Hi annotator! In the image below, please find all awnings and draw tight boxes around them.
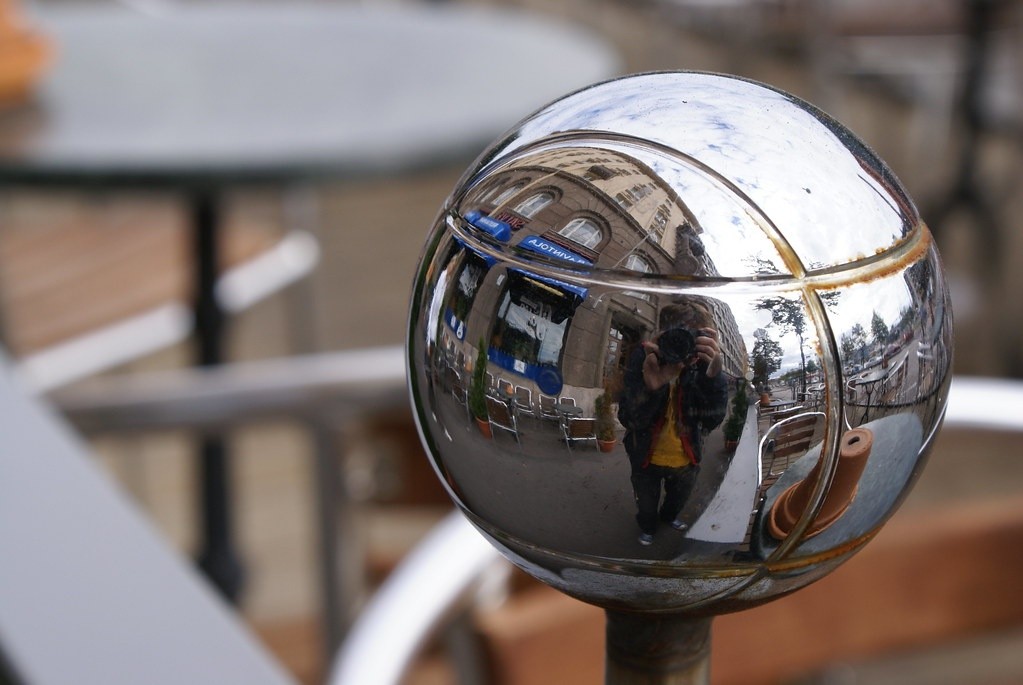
[506,234,592,303]
[454,210,512,269]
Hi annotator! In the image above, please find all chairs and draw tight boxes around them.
[427,323,598,456]
[751,353,909,515]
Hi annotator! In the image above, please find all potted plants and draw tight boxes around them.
[596,392,620,451]
[722,385,749,449]
[469,335,493,439]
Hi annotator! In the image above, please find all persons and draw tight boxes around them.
[616,303,728,545]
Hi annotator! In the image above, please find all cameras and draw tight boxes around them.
[657,327,710,358]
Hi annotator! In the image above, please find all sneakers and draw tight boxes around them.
[670,518,688,531]
[639,532,655,546]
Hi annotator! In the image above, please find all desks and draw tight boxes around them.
[766,400,797,410]
[855,360,897,423]
